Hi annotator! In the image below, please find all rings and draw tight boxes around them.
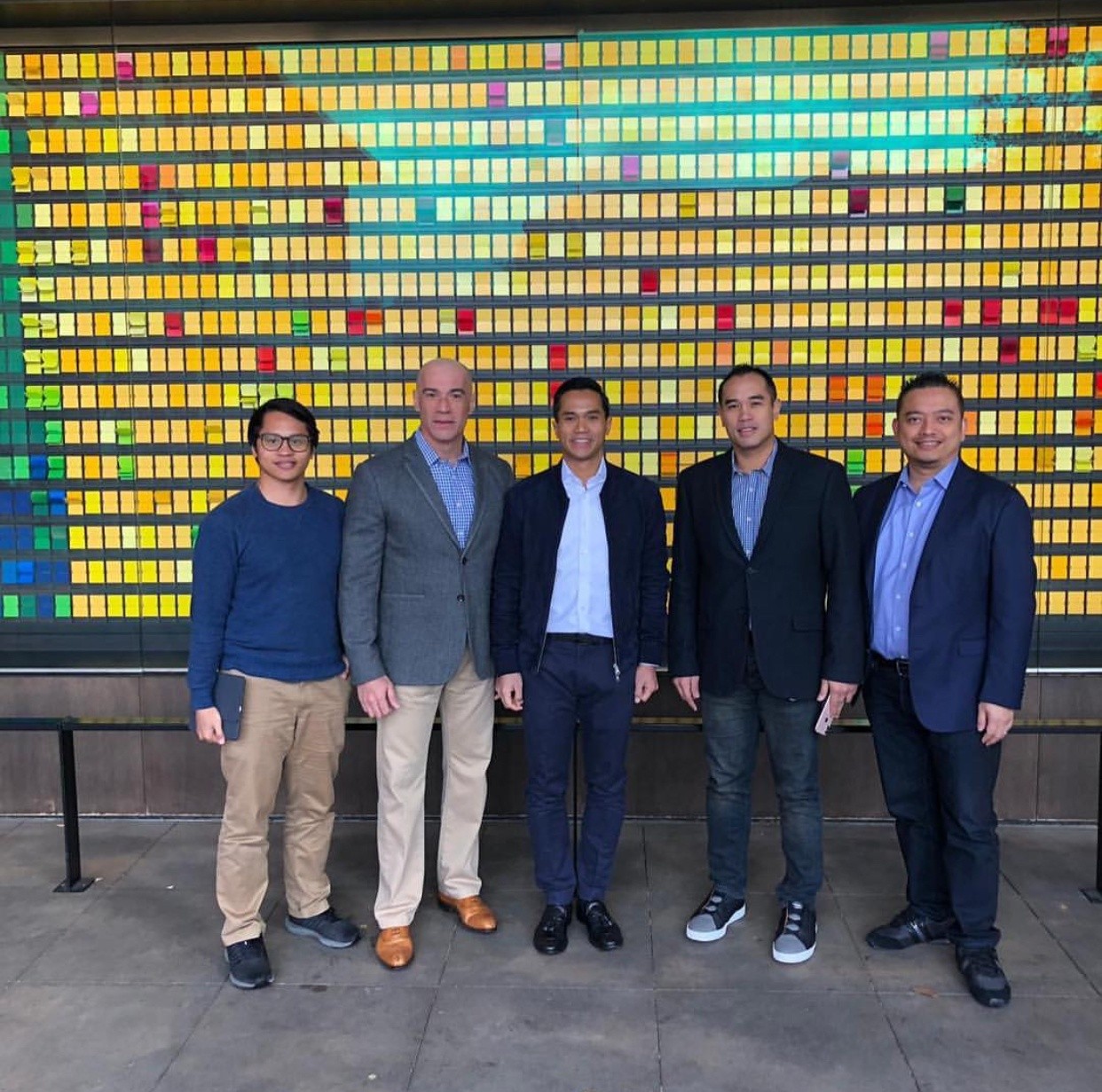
[1004,731,1007,735]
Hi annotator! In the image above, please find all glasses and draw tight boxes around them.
[256,433,313,453]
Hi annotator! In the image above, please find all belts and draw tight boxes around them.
[872,651,911,677]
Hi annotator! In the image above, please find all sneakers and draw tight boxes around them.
[956,944,1009,1008]
[867,910,960,949]
[223,938,274,990]
[686,887,747,941]
[284,907,360,949]
[772,900,819,962]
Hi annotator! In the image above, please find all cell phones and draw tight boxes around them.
[814,692,834,736]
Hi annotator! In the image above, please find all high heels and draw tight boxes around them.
[533,902,571,955]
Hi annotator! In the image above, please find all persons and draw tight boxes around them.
[852,372,1038,1008]
[665,364,865,964]
[337,357,516,968]
[487,377,668,954]
[187,398,361,989]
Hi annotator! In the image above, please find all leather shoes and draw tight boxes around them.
[374,924,412,969]
[438,890,496,933]
[576,899,622,950]
